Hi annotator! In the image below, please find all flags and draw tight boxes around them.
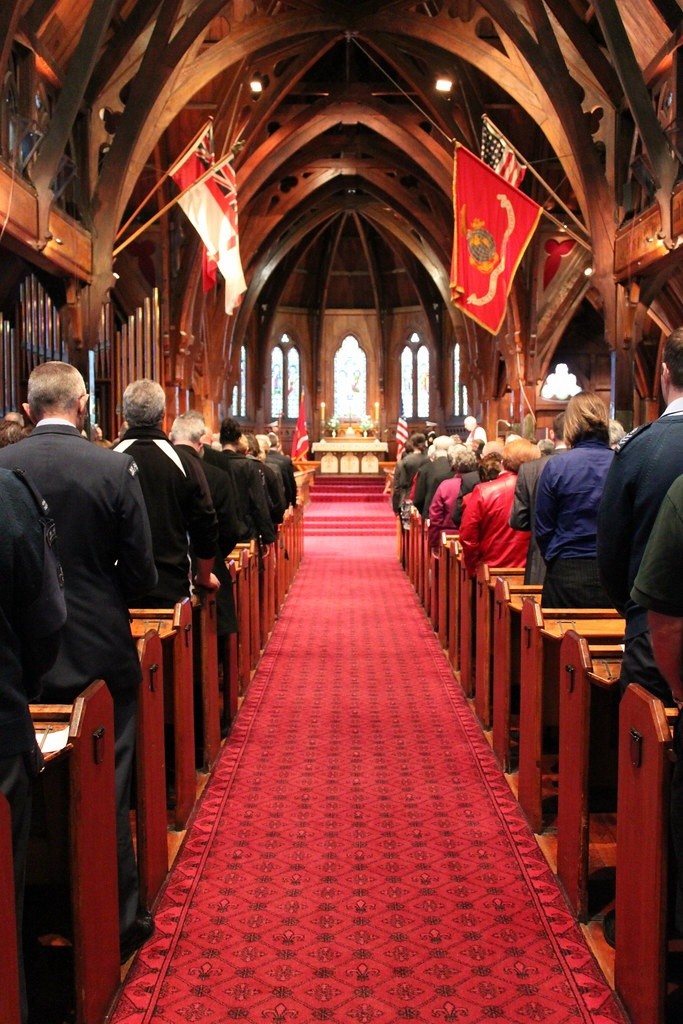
[169,123,217,295]
[481,128,527,187]
[176,154,247,314]
[451,144,544,336]
[396,396,409,459]
[291,394,308,471]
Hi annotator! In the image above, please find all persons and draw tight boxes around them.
[464,416,487,445]
[393,323,683,1024]
[0,360,300,1024]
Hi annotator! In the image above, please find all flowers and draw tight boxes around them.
[326,417,340,430]
[359,414,374,431]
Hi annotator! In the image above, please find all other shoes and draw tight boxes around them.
[120,905,155,965]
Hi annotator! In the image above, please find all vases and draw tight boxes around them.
[363,430,368,438]
[345,427,355,437]
[331,429,336,438]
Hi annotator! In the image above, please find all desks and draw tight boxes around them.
[0,495,683,1024]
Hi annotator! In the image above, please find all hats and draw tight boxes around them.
[269,421,278,427]
[425,421,437,427]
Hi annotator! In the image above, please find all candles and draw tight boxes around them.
[375,402,380,420]
[321,402,325,419]
